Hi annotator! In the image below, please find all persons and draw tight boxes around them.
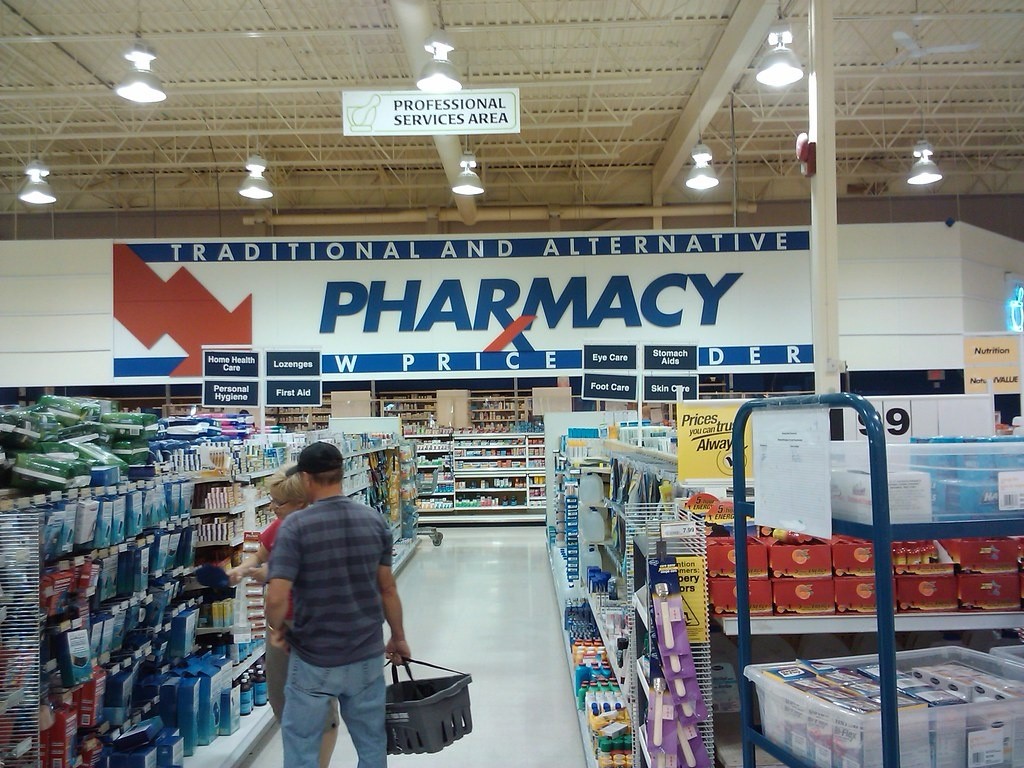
[265,441,410,768]
[226,462,340,767]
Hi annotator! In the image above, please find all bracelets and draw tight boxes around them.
[267,624,275,632]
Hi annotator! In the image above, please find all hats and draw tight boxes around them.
[287,441,344,477]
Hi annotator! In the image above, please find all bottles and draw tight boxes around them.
[212,633,227,661]
[248,669,256,682]
[254,670,267,706]
[572,637,633,768]
[240,679,251,716]
[226,632,252,666]
[254,665,266,678]
[242,673,249,679]
[244,676,254,711]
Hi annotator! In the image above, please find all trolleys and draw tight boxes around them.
[418,469,443,546]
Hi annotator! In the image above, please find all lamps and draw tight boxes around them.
[18,0,942,206]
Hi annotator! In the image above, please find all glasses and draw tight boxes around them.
[267,493,294,509]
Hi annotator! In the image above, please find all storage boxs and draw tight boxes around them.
[0,393,1024,768]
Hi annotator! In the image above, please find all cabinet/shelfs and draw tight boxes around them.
[732,391,1024,768]
[545,410,1024,768]
[382,389,527,429]
[451,433,546,515]
[404,433,454,515]
[266,394,383,428]
[0,417,422,768]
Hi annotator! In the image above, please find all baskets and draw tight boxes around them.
[383,657,473,755]
